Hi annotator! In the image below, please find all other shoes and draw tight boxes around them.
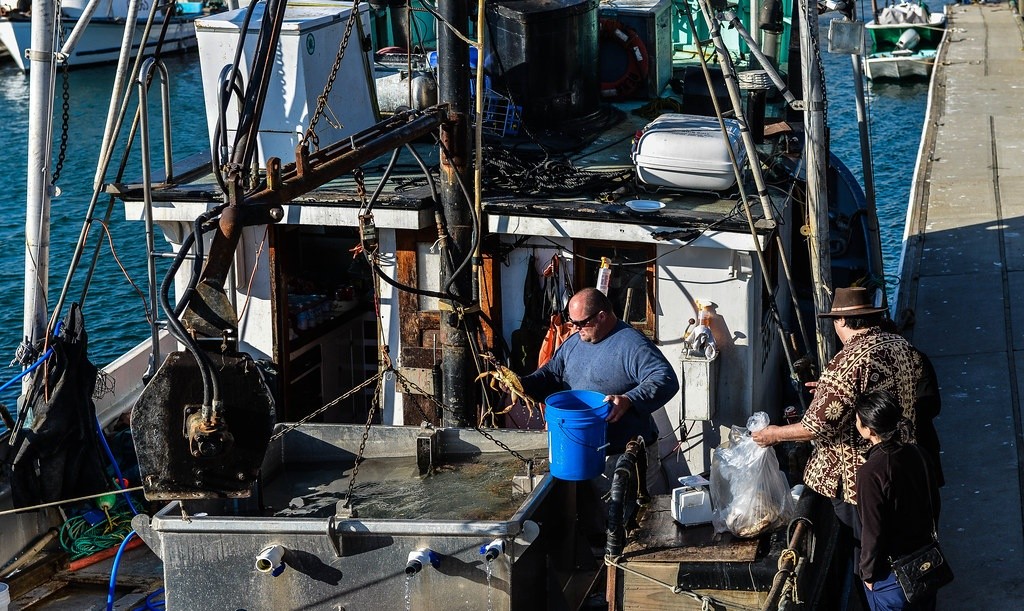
[590,547,619,557]
[587,589,622,606]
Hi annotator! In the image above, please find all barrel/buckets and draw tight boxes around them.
[0,582,10,611]
[544,389,611,480]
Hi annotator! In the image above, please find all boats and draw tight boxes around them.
[0,0,228,72]
[861,48,939,79]
[864,0,947,54]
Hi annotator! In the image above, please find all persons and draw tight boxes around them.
[499,287,680,556]
[753,287,946,610]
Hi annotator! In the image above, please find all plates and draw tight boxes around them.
[625,200,666,212]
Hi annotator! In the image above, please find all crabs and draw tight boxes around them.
[726,489,790,539]
[475,351,536,425]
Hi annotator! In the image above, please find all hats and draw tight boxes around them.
[818,287,888,318]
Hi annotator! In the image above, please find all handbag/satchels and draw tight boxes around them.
[709,411,795,538]
[891,540,954,603]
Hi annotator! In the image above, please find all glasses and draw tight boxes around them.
[572,312,599,330]
